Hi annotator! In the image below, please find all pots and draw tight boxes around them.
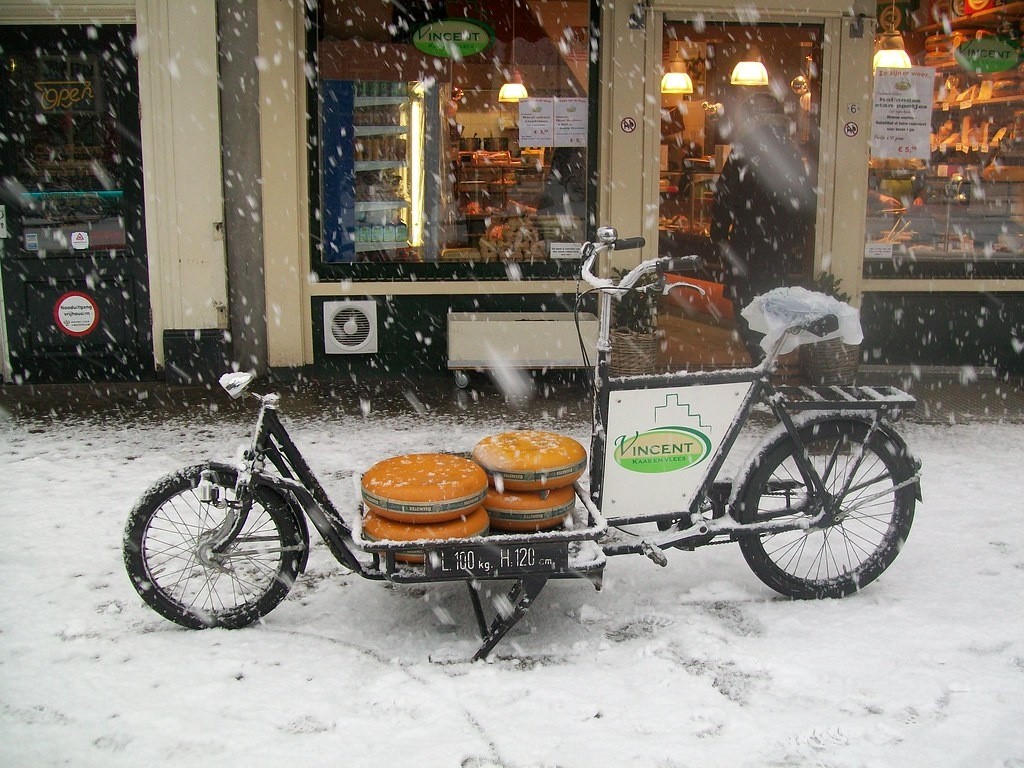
[484,138,508,151]
[460,137,480,151]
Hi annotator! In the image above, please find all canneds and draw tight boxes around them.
[353,79,409,161]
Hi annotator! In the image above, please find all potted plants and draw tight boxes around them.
[775,275,863,386]
[603,266,667,377]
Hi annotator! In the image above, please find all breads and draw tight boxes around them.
[479,217,547,262]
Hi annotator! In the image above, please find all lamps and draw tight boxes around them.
[872,0,912,69]
[498,0,527,102]
[661,39,694,94]
[731,47,768,86]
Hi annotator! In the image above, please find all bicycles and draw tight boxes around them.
[122,227,924,667]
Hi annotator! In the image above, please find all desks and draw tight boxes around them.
[688,173,721,228]
[871,163,1024,252]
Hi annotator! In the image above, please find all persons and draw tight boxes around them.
[708,92,811,368]
[534,146,586,313]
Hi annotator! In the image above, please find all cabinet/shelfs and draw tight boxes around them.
[914,0,1024,158]
[453,163,545,247]
[353,96,411,252]
